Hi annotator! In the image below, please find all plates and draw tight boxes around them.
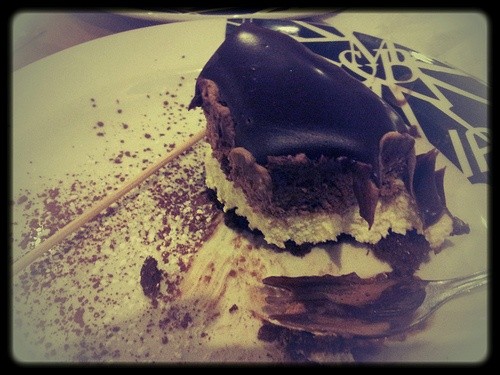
[13,17,488,363]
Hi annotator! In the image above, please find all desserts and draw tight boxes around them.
[186,23,468,362]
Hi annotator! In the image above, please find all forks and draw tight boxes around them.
[261,271,487,339]
